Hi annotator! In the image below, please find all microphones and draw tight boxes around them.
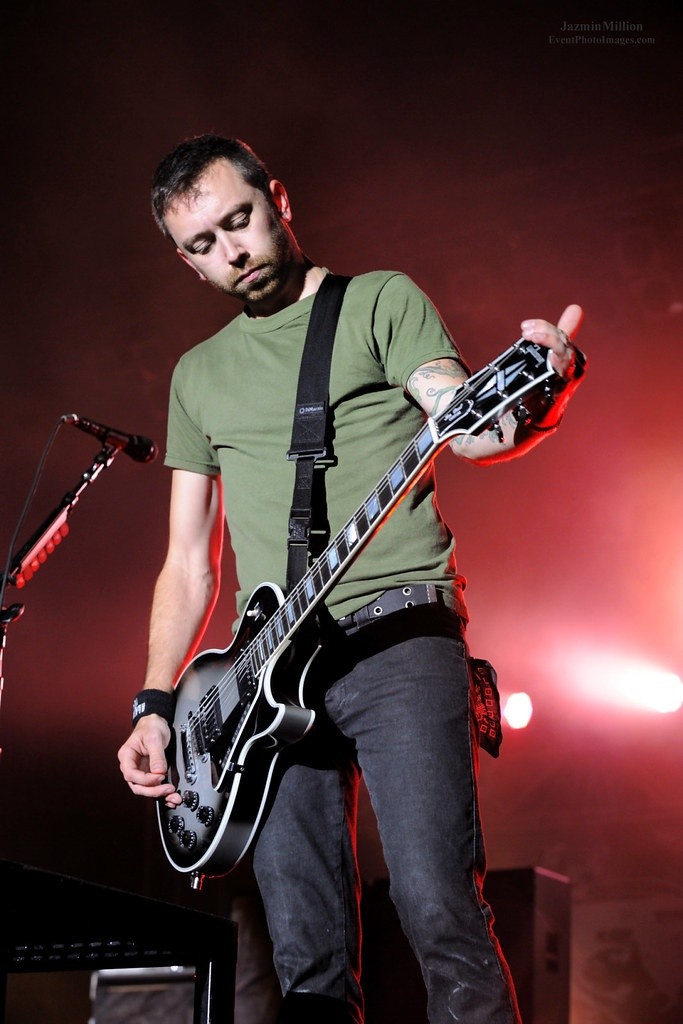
[60,413,159,463]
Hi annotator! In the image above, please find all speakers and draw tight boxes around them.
[365,865,573,1024]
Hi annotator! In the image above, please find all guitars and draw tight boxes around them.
[156,336,559,881]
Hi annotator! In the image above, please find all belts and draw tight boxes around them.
[336,584,438,629]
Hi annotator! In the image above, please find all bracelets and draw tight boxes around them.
[529,416,564,433]
[130,689,174,725]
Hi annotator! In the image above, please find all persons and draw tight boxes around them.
[117,131,590,1024]
